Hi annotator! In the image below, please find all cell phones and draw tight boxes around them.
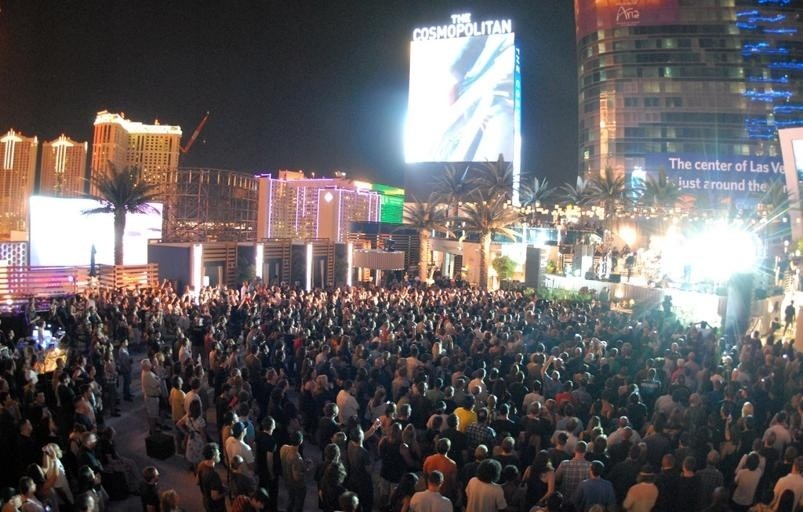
[376,418,380,425]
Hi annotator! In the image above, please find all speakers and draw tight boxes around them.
[756,289,767,299]
[585,272,599,280]
[682,282,728,297]
[608,274,621,283]
[772,287,783,295]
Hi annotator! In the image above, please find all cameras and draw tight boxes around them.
[45,450,50,455]
